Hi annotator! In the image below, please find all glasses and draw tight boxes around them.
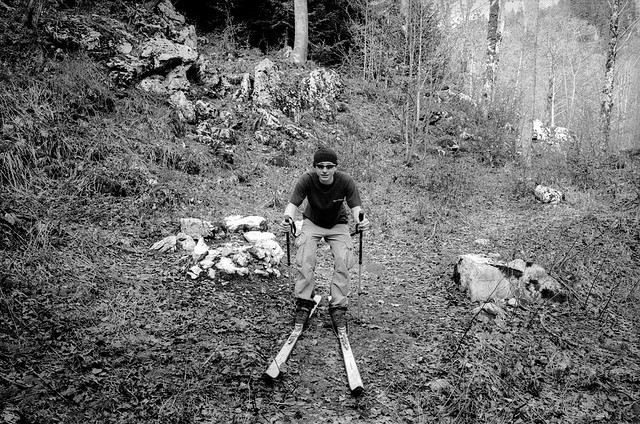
[315,164,334,170]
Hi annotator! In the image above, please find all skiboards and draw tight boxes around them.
[263,295,366,393]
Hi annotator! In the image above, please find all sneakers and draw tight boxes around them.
[330,306,347,327]
[295,306,310,324]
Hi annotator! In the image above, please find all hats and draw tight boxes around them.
[313,149,338,167]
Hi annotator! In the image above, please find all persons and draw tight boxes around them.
[281,148,369,330]
